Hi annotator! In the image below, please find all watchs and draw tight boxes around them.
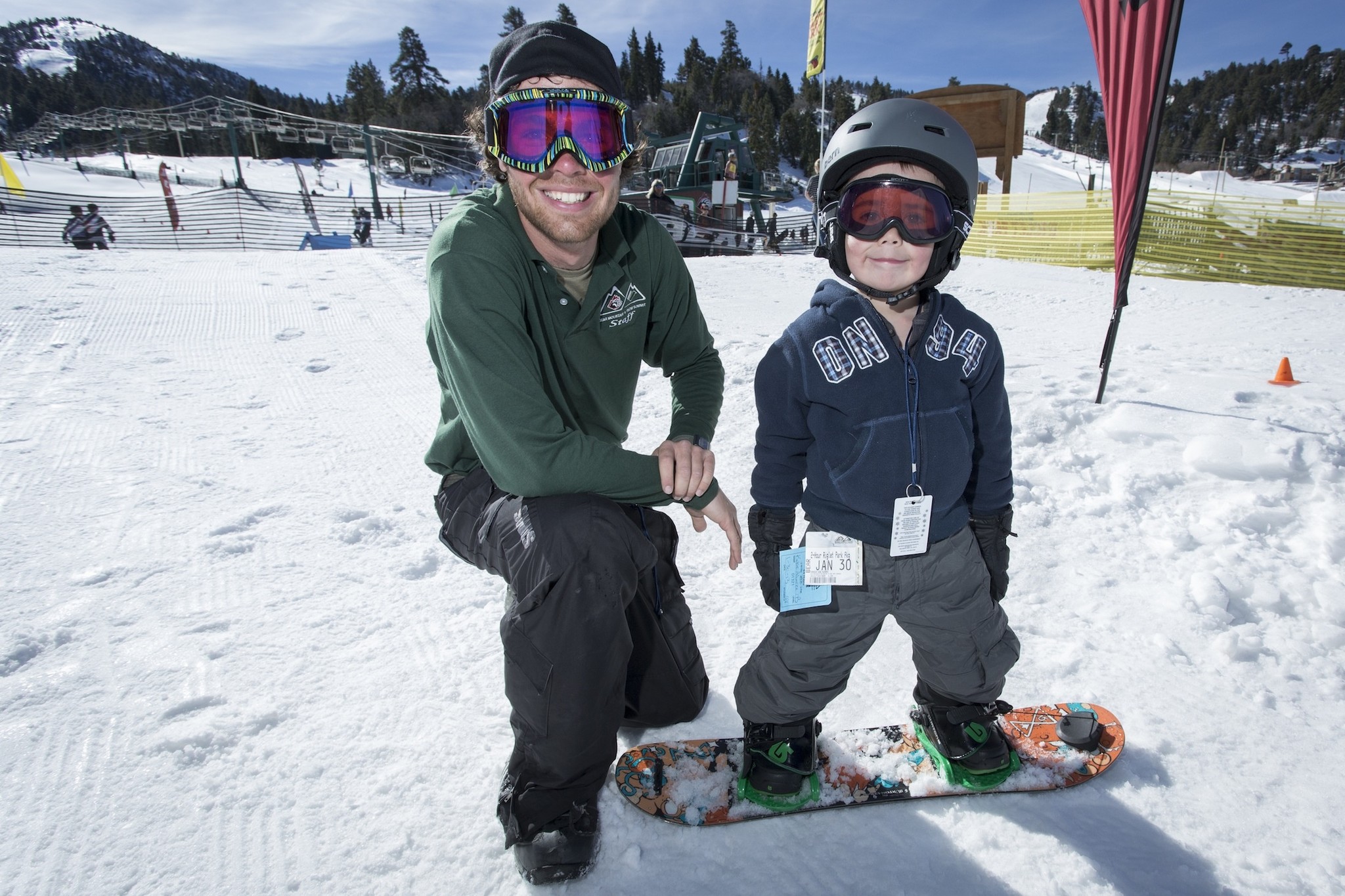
[671,435,710,450]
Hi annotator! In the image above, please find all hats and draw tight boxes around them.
[750,211,754,216]
[487,20,633,117]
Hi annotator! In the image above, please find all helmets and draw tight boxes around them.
[815,98,978,277]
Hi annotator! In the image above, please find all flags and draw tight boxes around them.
[1080,0,1171,312]
[348,182,353,198]
[399,199,403,217]
[159,161,179,231]
[806,0,826,78]
[0,152,26,198]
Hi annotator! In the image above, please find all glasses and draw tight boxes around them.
[702,208,709,211]
[836,177,955,245]
[483,90,636,173]
[654,185,663,188]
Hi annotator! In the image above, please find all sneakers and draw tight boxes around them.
[917,691,1010,775]
[743,714,812,795]
[500,771,601,885]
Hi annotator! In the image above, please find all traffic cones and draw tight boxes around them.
[1268,357,1300,386]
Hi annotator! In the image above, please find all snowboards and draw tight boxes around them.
[613,701,1127,826]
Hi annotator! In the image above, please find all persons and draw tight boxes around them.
[351,207,371,244]
[746,210,755,249]
[645,179,715,256]
[805,158,825,245]
[789,224,809,245]
[426,19,741,887]
[0,200,7,214]
[766,213,781,253]
[83,203,116,250]
[724,152,738,179]
[386,203,393,221]
[62,206,92,250]
[734,95,1021,812]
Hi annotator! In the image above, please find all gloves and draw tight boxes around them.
[746,505,792,611]
[968,511,1013,602]
